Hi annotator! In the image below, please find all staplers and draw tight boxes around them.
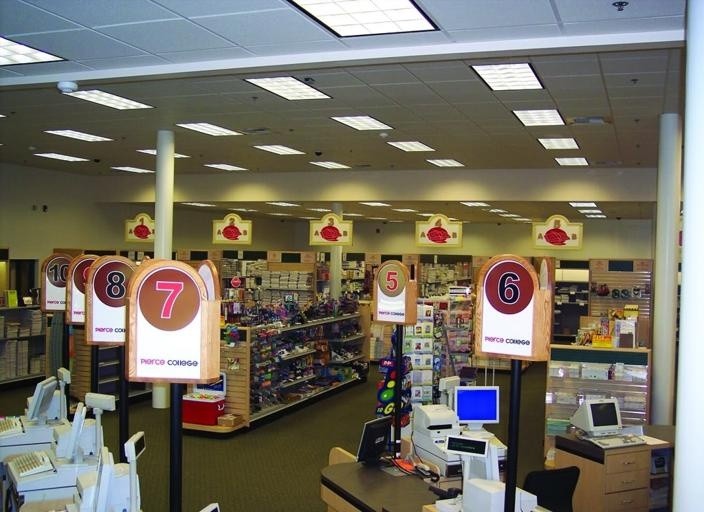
[416,462,431,476]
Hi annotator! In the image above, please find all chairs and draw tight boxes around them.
[521,465,580,512]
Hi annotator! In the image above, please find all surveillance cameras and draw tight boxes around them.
[93,157,101,163]
[497,222,502,226]
[616,216,621,220]
[314,151,322,156]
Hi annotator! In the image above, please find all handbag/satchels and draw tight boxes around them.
[597,284,609,296]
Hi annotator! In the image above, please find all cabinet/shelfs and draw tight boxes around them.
[543,344,651,461]
[1,250,591,386]
[182,302,372,434]
[52,311,152,417]
[553,432,650,511]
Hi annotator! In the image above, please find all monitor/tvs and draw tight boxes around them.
[356,416,393,466]
[570,399,622,437]
[464,444,499,482]
[61,402,89,467]
[91,446,114,512]
[26,376,58,427]
[454,385,500,433]
